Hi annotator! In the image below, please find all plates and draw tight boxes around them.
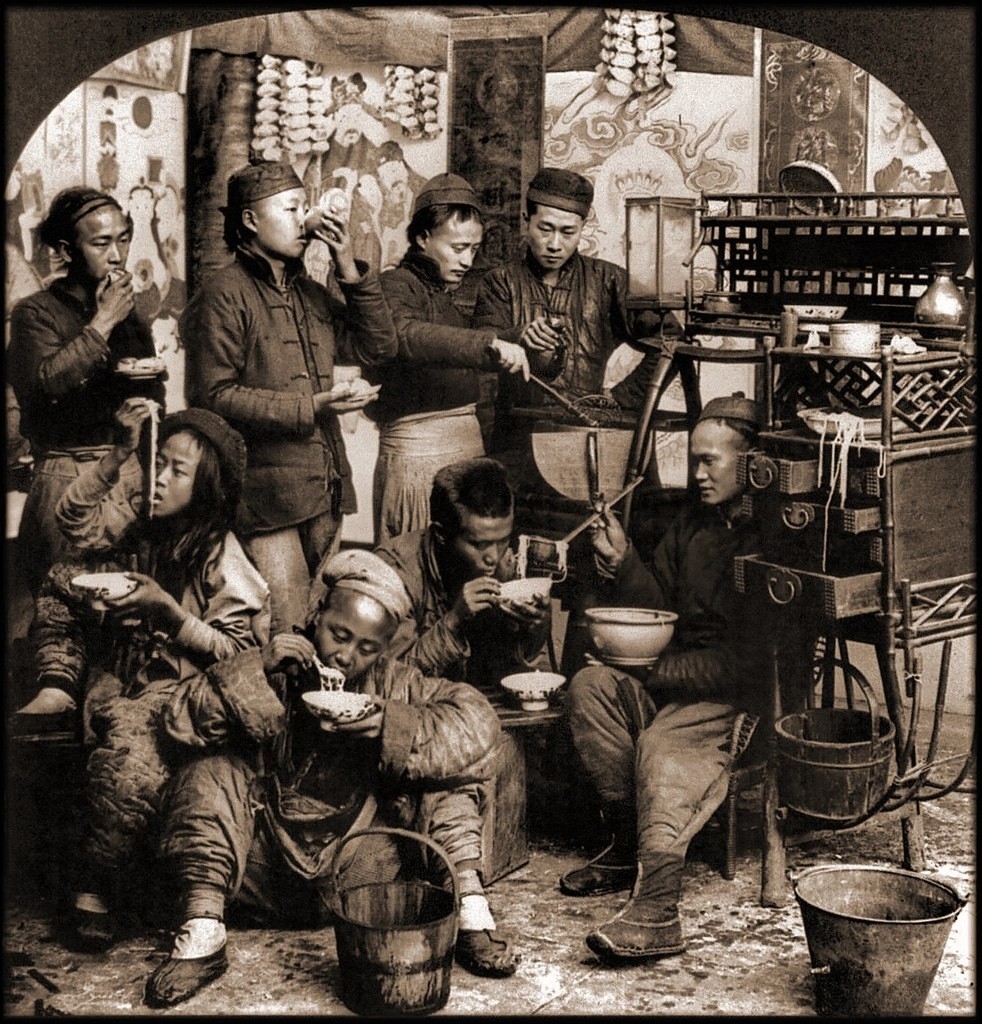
[779,160,845,219]
[115,370,164,379]
[344,384,382,402]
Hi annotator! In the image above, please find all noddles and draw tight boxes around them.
[309,667,365,710]
[144,399,159,518]
[516,535,568,584]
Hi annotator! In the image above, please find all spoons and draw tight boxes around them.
[312,655,345,680]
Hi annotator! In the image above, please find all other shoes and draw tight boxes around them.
[7,706,81,741]
[456,925,518,979]
[68,906,116,951]
[145,943,228,1006]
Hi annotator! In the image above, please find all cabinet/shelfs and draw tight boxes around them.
[622,191,978,908]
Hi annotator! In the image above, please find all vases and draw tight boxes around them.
[913,262,969,337]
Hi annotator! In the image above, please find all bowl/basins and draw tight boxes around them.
[784,305,847,332]
[703,293,742,321]
[830,324,881,355]
[492,577,553,606]
[585,608,679,665]
[797,407,921,434]
[302,692,375,731]
[72,572,138,610]
[500,672,565,710]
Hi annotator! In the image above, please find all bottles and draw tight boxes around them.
[916,262,967,339]
[526,317,565,385]
[782,313,797,347]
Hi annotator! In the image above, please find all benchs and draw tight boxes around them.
[8,680,568,884]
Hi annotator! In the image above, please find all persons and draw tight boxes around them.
[470,168,685,457]
[145,550,522,1008]
[178,160,383,635]
[368,458,552,678]
[360,171,529,539]
[561,392,825,958]
[0,397,273,944]
[8,186,168,599]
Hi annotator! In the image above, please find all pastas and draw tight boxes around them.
[807,409,888,572]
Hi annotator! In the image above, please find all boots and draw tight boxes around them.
[586,850,686,957]
[560,799,638,897]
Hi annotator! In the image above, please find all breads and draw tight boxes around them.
[116,357,164,372]
[305,206,341,239]
[108,268,128,284]
[331,378,373,399]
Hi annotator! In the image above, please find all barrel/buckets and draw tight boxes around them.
[330,828,461,1016]
[776,658,896,821]
[793,864,970,1016]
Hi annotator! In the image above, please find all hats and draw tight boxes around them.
[414,172,482,213]
[527,168,593,218]
[40,185,120,239]
[158,408,246,490]
[322,549,412,623]
[228,145,304,204]
[697,391,765,426]
